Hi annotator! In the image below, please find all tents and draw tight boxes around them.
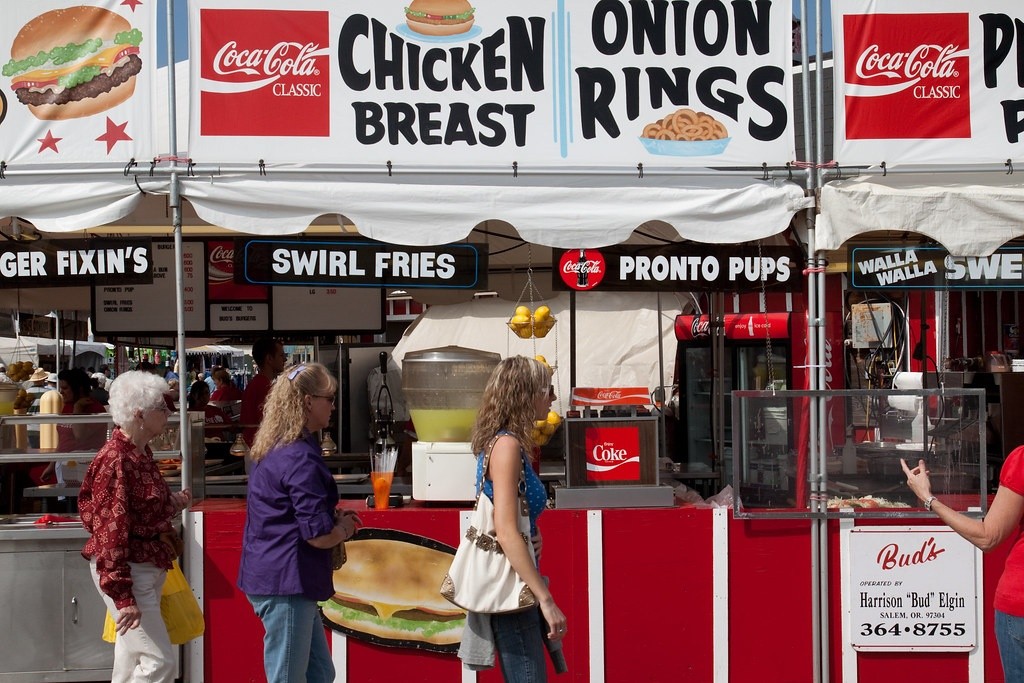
[0,336,105,385]
[368,290,699,473]
[185,345,244,374]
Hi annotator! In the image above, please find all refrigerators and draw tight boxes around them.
[675,311,805,508]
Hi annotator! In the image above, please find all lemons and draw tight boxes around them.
[535,355,548,363]
[511,306,554,338]
[7,360,36,408]
[531,411,561,443]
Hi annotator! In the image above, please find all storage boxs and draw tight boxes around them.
[763,406,788,446]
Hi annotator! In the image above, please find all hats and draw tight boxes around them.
[27,367,50,382]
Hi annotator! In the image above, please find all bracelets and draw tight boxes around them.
[925,496,937,512]
[338,524,349,538]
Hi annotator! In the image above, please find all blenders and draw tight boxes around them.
[401,346,500,504]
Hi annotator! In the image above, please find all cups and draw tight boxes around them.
[371,472,393,509]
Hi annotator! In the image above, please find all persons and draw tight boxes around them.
[241,339,288,474]
[0,361,243,513]
[900,445,1024,683]
[78,370,192,683]
[471,355,566,683]
[236,362,360,683]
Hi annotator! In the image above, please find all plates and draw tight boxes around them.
[366,495,412,506]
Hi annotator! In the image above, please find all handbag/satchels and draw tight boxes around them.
[441,434,538,612]
[102,557,205,645]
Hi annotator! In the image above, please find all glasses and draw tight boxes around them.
[533,385,556,397]
[308,395,337,404]
[144,402,169,415]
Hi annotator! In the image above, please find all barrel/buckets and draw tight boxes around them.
[763,406,788,444]
[750,463,780,485]
[770,362,786,380]
[0,385,17,415]
[723,447,732,483]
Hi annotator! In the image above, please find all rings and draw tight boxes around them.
[560,629,562,632]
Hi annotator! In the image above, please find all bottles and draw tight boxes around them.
[859,443,881,448]
[11,382,30,408]
[142,349,177,366]
[751,362,766,390]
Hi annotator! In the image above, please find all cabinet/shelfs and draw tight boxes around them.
[0,536,180,683]
[0,411,207,504]
